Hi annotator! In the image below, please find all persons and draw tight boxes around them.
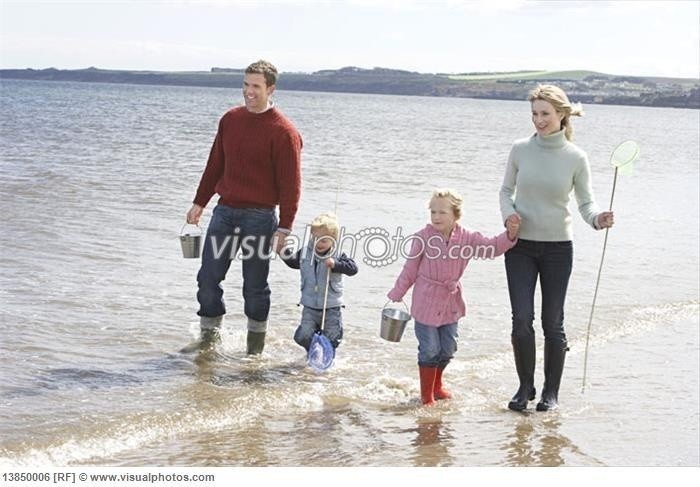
[387,188,522,406]
[499,85,615,411]
[272,212,358,357]
[179,60,303,356]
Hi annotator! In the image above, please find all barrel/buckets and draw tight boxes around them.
[179,219,206,258]
[381,299,413,343]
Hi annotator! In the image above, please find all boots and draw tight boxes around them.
[509,330,570,411]
[298,340,312,352]
[247,318,268,355]
[420,367,452,405]
[331,338,342,348]
[180,315,223,354]
